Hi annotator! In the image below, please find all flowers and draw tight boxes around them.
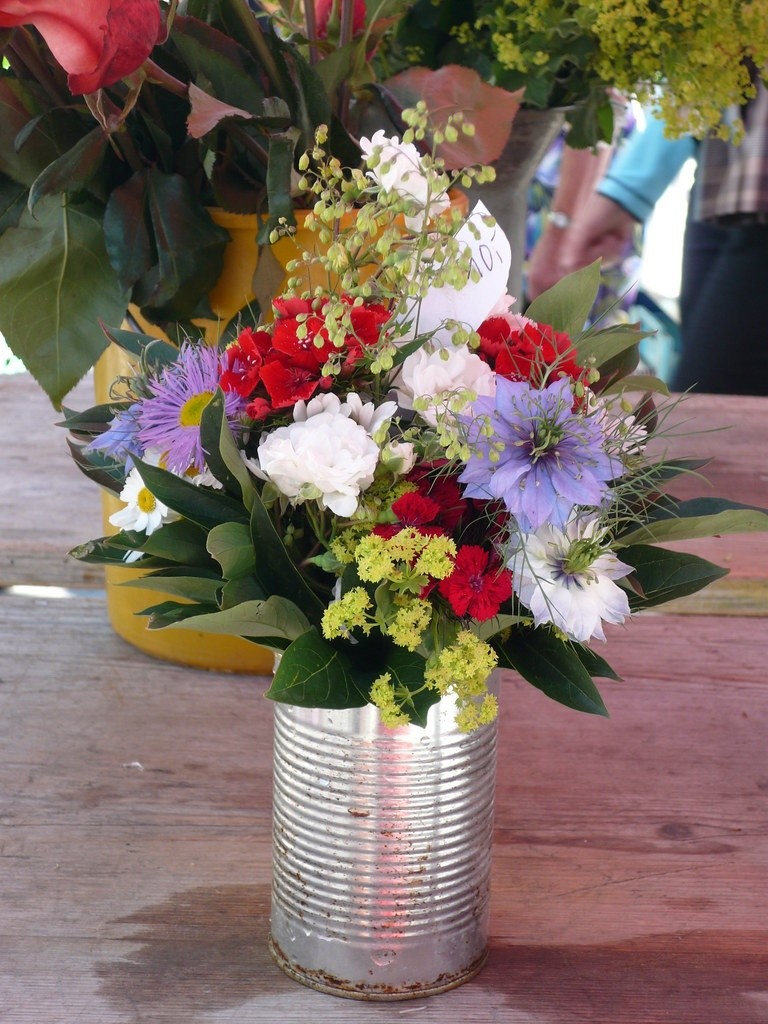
[0,0,765,416]
[61,112,739,729]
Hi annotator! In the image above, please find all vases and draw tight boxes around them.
[267,649,491,1006]
[92,172,471,674]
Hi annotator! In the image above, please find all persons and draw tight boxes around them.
[520,68,768,397]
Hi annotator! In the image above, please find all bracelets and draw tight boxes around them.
[546,210,569,229]
